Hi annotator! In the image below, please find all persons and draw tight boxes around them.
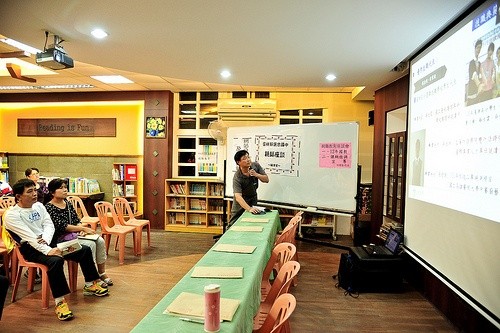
[2,179,109,320]
[24,167,51,205]
[466,40,500,105]
[45,178,112,288]
[229,150,269,228]
[411,140,423,186]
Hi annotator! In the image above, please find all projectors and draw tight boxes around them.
[34,52,74,70]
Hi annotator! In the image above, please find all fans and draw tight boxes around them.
[208,120,227,145]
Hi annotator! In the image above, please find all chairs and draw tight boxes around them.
[251,211,304,333]
[0,196,150,310]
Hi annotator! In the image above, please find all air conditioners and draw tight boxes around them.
[217,98,277,121]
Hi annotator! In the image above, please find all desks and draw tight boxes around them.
[67,192,105,217]
[130,210,282,333]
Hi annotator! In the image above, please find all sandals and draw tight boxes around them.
[97,271,112,286]
[95,279,108,288]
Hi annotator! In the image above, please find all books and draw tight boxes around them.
[189,184,206,195]
[168,213,223,227]
[78,233,99,241]
[170,184,185,195]
[163,243,256,321]
[210,184,224,196]
[209,200,223,211]
[241,218,269,223]
[57,239,82,256]
[39,176,101,194]
[231,226,263,231]
[274,203,332,225]
[112,165,137,197]
[190,199,206,210]
[169,198,185,209]
[0,155,9,184]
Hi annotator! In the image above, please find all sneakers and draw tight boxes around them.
[55,300,73,321]
[82,284,108,297]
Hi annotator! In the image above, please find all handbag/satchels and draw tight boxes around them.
[56,232,77,241]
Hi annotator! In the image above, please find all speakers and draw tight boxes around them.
[369,110,374,126]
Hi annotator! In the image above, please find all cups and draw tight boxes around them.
[204,283,221,333]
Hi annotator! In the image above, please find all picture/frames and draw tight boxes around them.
[145,117,167,139]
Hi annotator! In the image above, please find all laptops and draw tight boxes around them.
[362,226,403,258]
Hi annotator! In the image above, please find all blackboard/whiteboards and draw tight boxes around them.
[224,121,359,215]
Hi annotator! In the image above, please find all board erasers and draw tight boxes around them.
[307,207,316,212]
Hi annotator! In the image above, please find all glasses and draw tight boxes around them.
[28,172,39,177]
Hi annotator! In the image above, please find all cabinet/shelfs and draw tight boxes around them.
[165,181,336,240]
[0,152,10,184]
[113,163,137,216]
